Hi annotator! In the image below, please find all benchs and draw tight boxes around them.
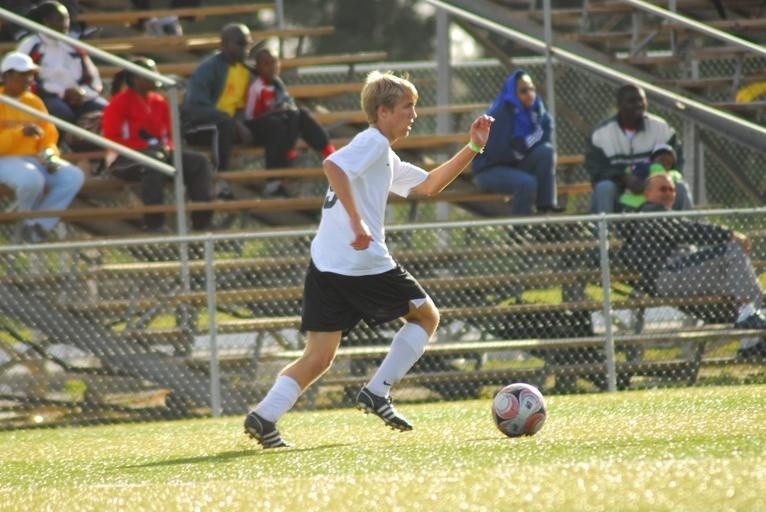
[0,0,766,408]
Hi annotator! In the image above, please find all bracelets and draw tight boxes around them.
[468,142,484,154]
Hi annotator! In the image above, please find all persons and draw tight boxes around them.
[585,85,695,249]
[243,71,495,449]
[470,70,563,214]
[2,1,339,241]
[620,173,766,358]
[615,144,683,213]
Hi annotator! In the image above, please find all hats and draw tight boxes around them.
[0,52,40,74]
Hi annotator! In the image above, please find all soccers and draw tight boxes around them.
[492,382,547,437]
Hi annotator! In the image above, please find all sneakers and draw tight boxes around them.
[737,341,765,359]
[243,411,291,449]
[734,312,765,330]
[355,386,412,433]
